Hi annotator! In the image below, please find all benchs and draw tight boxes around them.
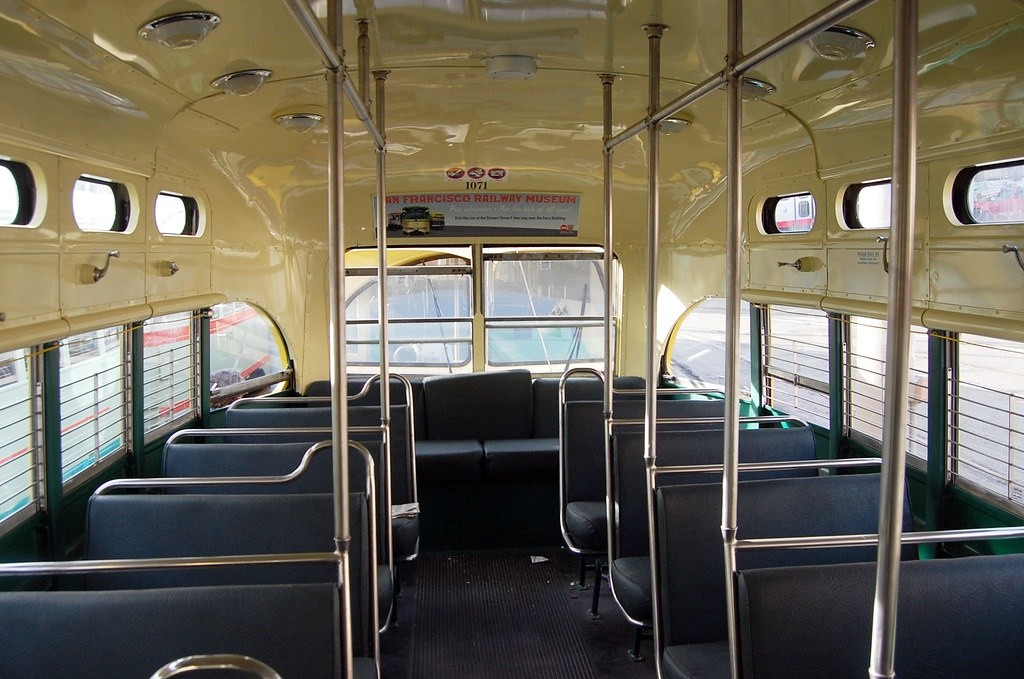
[561,369,1024,679]
[0,373,420,679]
[300,370,651,527]
